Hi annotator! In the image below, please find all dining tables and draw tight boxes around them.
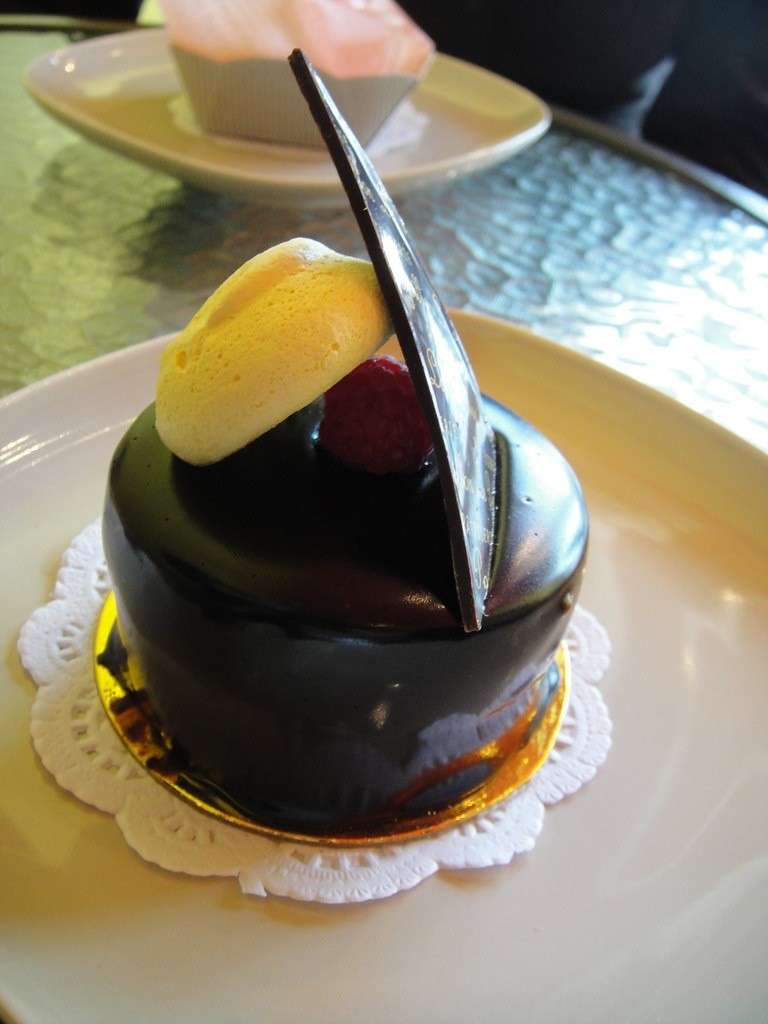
[0,16,767,457]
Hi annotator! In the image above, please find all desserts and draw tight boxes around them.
[103,237,591,833]
[157,0,434,149]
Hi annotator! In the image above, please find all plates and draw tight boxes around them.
[4,309,767,1023]
[29,29,560,211]
